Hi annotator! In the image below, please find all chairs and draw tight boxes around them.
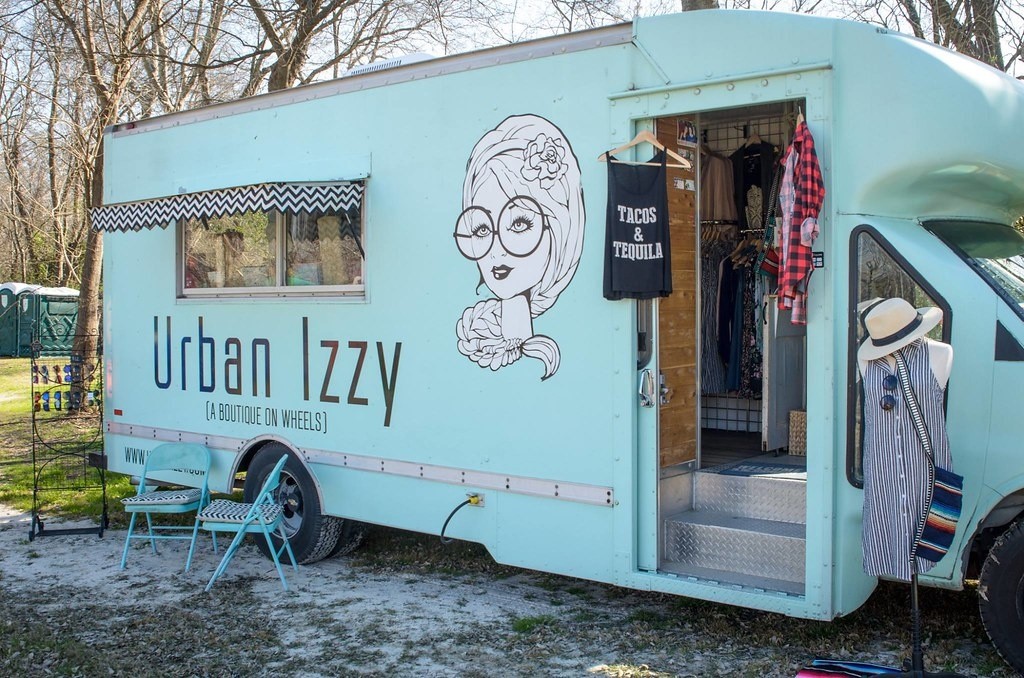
[118,441,220,570]
[196,454,300,592]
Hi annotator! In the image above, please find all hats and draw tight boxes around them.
[860,297,890,348]
[858,297,943,361]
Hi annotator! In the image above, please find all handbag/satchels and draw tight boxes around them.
[913,467,964,563]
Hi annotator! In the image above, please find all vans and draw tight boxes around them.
[86,7,1023,677]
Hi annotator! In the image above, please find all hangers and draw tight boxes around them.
[597,103,821,273]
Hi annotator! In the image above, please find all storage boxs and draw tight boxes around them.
[240,263,277,288]
[285,259,324,285]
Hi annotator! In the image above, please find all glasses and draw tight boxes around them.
[42,391,50,411]
[880,375,897,410]
[34,392,41,412]
[31,365,39,384]
[41,365,49,384]
[53,365,62,383]
[73,365,81,383]
[63,364,72,382]
[54,391,62,411]
[93,390,103,406]
[87,364,95,382]
[72,392,80,410]
[63,391,73,411]
[87,390,93,406]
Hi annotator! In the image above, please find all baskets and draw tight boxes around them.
[788,410,807,456]
